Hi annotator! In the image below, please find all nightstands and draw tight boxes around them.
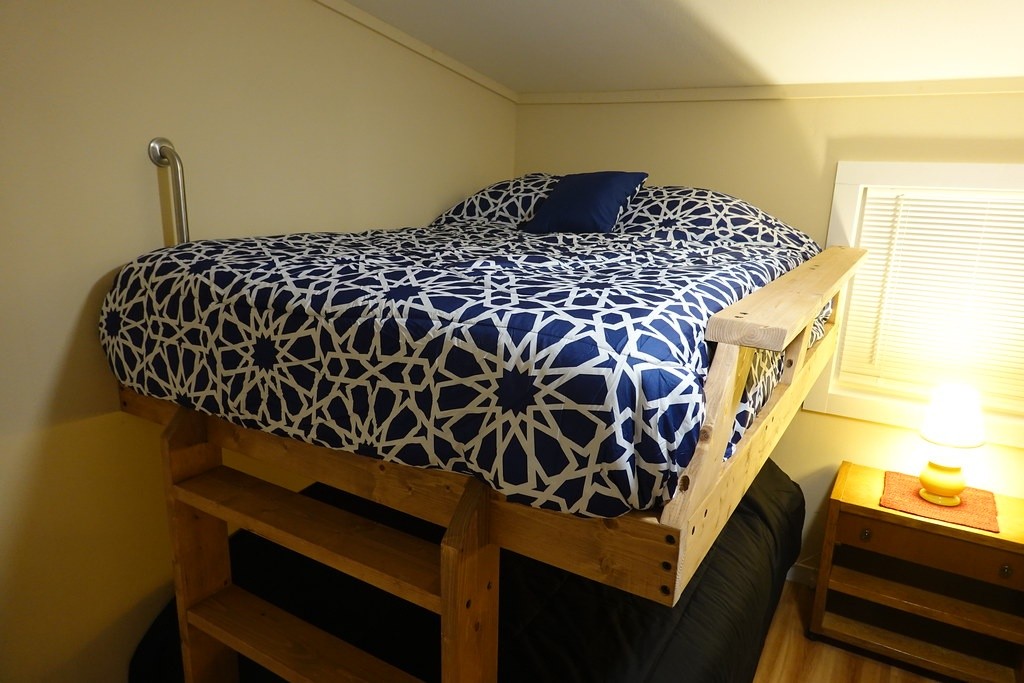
[807,455,1024,682]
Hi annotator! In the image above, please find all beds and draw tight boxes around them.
[99,186,868,683]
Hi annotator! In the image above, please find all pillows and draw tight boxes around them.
[519,170,649,234]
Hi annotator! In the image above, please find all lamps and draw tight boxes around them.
[919,373,989,506]
